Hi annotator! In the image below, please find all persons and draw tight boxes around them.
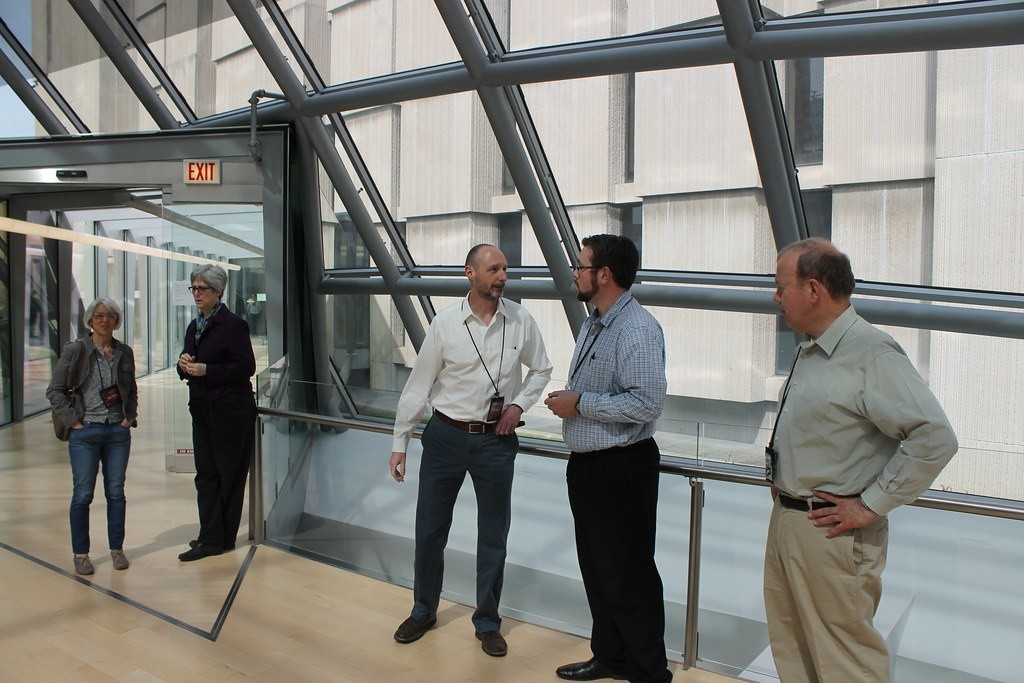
[45,296,138,575]
[176,264,256,561]
[236,290,245,318]
[763,238,960,683]
[247,293,262,336]
[388,244,554,657]
[544,233,674,683]
[29,290,43,339]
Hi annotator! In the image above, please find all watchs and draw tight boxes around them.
[575,394,582,415]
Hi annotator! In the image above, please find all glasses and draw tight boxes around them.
[92,314,118,321]
[576,262,612,275]
[188,287,212,294]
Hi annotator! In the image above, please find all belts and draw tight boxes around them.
[778,491,837,512]
[434,409,525,434]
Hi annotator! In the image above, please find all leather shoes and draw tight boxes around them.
[556,657,627,680]
[475,630,507,656]
[393,616,436,643]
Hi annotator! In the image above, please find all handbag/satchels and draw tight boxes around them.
[52,340,86,441]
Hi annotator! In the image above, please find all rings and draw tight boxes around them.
[191,372,192,375]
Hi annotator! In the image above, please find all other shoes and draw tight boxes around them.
[179,539,236,561]
[110,549,129,570]
[74,553,94,574]
